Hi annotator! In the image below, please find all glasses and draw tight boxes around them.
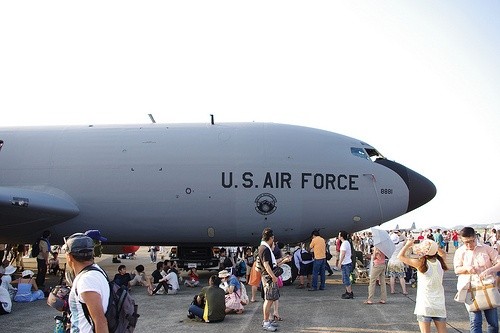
[463,237,476,243]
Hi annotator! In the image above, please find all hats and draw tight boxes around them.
[420,240,438,255]
[4,265,17,274]
[22,270,35,278]
[62,233,95,256]
[85,230,109,241]
[218,270,231,277]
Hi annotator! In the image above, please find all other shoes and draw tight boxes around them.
[306,283,311,288]
[296,284,305,289]
[274,316,283,321]
[270,320,278,327]
[307,288,316,291]
[333,266,338,270]
[341,290,353,299]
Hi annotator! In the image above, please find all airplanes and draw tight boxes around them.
[0,113,438,287]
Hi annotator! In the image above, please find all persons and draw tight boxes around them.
[0,231,63,315]
[189,275,225,323]
[258,228,292,332]
[309,230,326,291]
[397,238,448,333]
[217,242,314,314]
[113,246,200,296]
[64,229,111,333]
[453,227,500,333]
[326,225,500,304]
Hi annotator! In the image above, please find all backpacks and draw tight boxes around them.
[234,282,250,306]
[191,295,205,308]
[31,239,47,258]
[75,266,140,333]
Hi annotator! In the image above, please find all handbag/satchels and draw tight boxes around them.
[327,253,332,261]
[463,272,500,312]
[301,253,312,261]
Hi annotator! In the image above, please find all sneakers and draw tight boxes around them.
[262,321,278,332]
